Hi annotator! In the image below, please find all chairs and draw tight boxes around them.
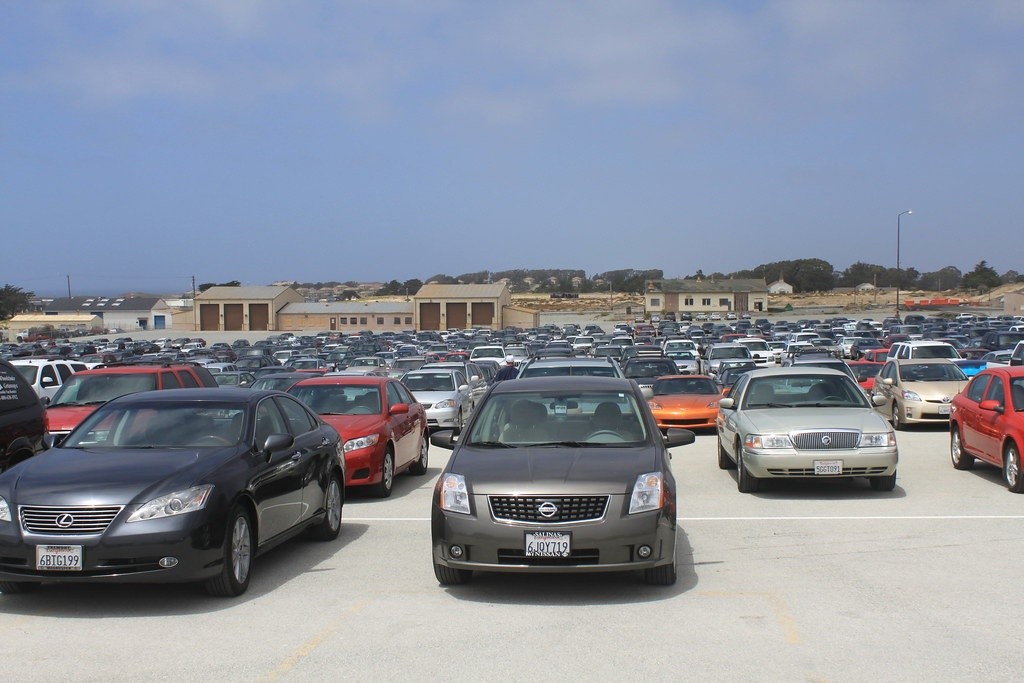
[498,400,550,441]
[357,391,379,412]
[319,393,346,411]
[753,384,774,402]
[584,401,634,442]
[804,384,829,402]
[221,412,244,442]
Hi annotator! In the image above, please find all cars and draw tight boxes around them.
[284,378,430,498]
[0,312,1024,428]
[875,362,970,431]
[714,365,899,491]
[428,376,697,587]
[950,365,1024,493]
[0,388,346,597]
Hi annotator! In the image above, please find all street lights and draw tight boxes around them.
[606,281,613,310]
[897,211,915,316]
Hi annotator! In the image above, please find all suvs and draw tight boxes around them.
[681,312,693,321]
[0,359,48,474]
[41,367,220,445]
[664,312,676,321]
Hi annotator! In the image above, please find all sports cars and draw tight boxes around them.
[645,374,729,430]
